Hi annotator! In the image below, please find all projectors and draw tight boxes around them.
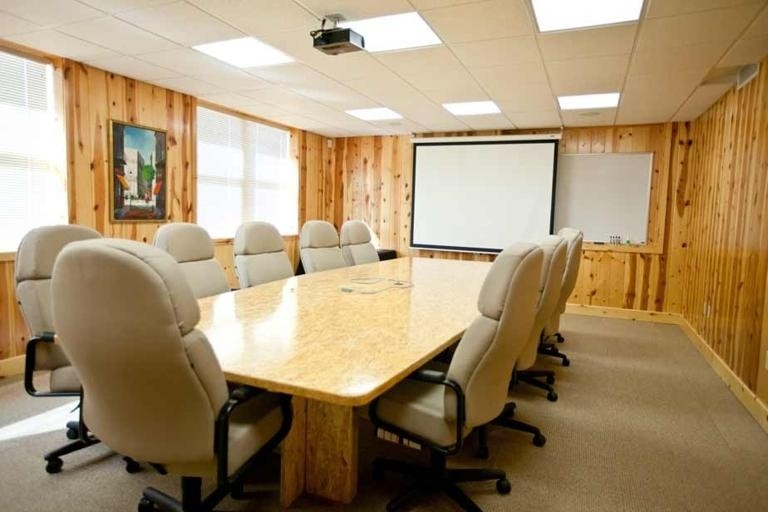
[310,29,365,56]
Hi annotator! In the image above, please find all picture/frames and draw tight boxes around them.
[108,119,171,223]
